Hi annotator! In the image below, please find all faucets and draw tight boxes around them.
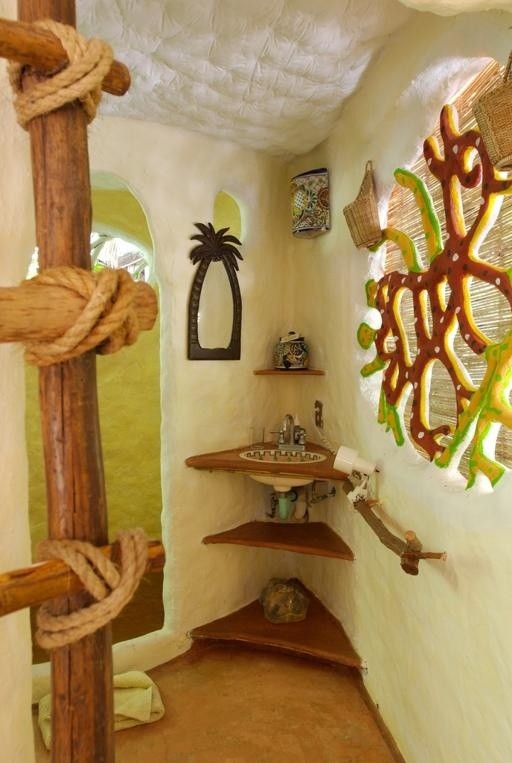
[281,414,295,444]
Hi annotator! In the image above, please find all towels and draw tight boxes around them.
[35,664,167,746]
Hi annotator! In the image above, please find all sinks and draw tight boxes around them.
[239,448,327,492]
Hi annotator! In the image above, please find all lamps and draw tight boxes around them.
[282,165,330,239]
[325,445,377,505]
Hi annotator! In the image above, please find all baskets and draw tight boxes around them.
[341,160,383,250]
[472,51,512,173]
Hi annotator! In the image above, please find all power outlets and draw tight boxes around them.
[311,400,324,429]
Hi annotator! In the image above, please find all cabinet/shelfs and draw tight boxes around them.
[182,367,364,673]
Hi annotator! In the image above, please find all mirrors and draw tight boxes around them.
[187,220,245,362]
